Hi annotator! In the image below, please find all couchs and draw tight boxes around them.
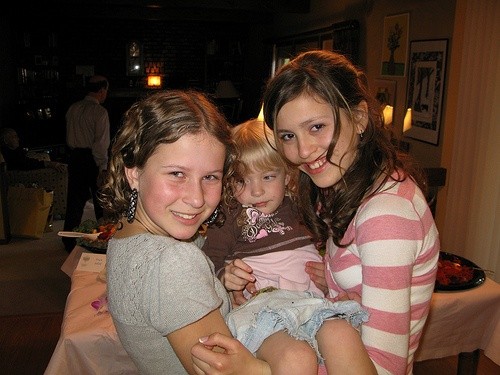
[0,153,68,231]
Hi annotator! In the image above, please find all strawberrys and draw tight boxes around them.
[433,258,476,285]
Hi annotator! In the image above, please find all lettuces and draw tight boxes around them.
[74,218,120,250]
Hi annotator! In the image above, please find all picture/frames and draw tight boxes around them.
[402,38,448,146]
[367,78,397,142]
[379,12,411,78]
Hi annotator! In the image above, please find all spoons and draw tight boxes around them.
[447,260,495,276]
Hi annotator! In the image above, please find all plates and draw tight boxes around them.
[432,249,486,294]
[79,221,111,252]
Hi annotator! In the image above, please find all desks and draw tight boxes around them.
[44,245,500,375]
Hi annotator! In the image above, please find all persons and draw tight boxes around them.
[2,129,65,171]
[200,119,380,374]
[97,89,362,375]
[191,49,441,375]
[62,75,111,252]
